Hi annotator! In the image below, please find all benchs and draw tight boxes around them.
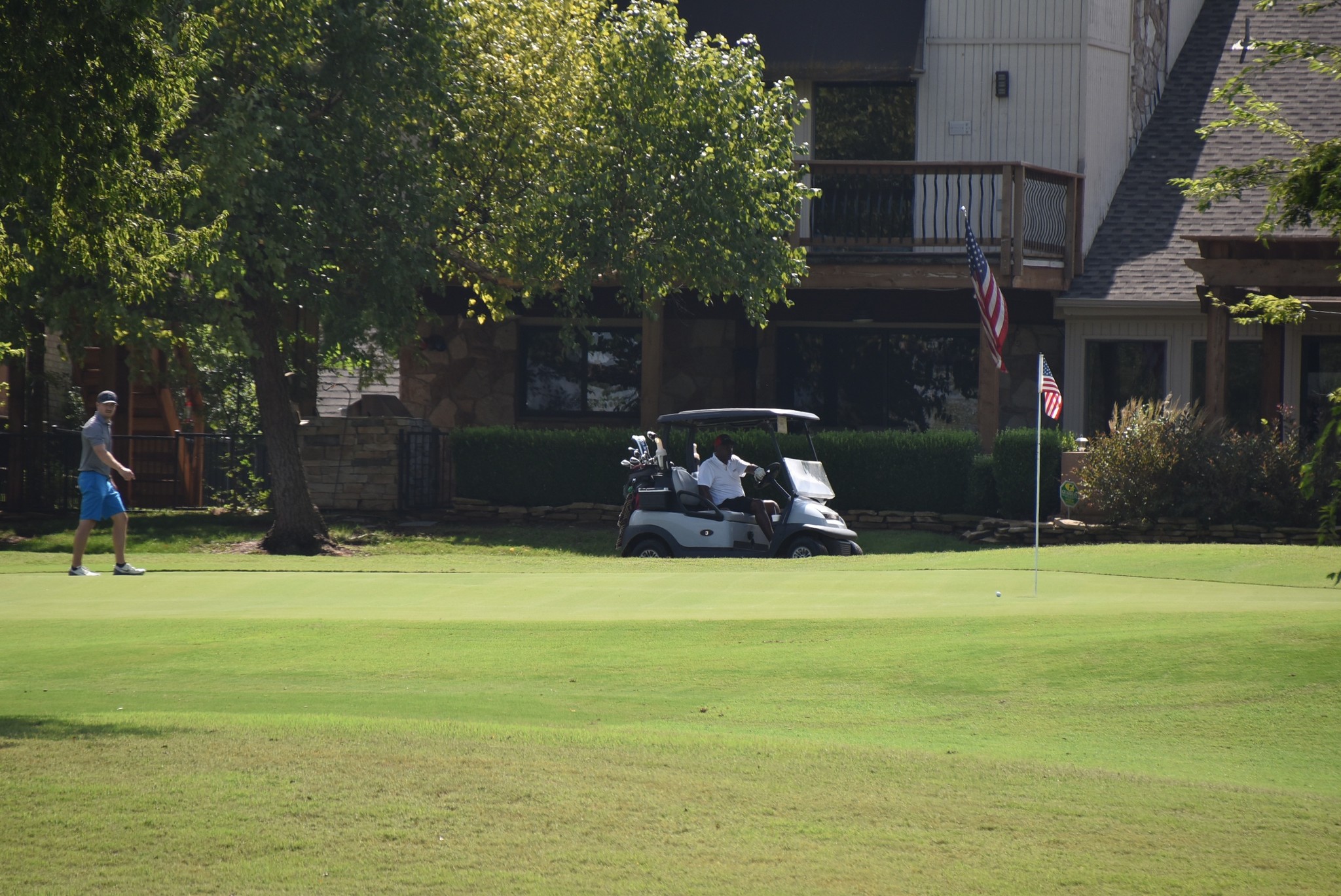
[672,469,758,525]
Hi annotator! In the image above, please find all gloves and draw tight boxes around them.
[753,466,766,482]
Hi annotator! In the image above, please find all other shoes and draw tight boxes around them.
[113,563,145,575]
[69,566,99,576]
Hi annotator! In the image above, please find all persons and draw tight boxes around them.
[68,390,146,576]
[698,434,780,542]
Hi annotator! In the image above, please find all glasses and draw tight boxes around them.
[103,403,116,406]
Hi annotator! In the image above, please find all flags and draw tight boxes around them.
[1038,353,1063,420]
[960,206,1008,374]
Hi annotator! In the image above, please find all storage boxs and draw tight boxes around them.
[638,486,672,510]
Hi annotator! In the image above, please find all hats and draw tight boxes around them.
[97,390,118,404]
[714,434,738,446]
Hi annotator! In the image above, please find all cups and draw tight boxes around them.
[772,515,781,522]
